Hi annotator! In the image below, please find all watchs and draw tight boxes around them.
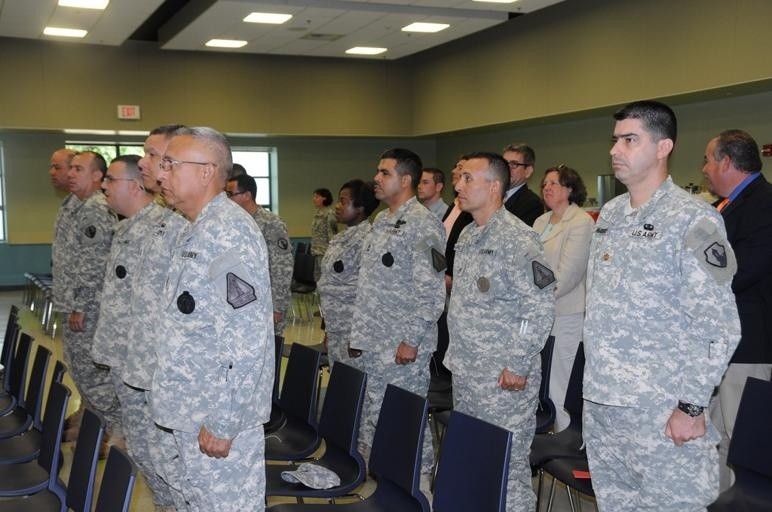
[677,399,703,417]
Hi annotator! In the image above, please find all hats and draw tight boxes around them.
[280,459,342,490]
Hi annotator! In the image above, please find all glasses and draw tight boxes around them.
[157,158,219,171]
[102,175,134,183]
[227,189,244,197]
[507,160,530,167]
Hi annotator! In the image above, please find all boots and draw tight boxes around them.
[61,400,111,460]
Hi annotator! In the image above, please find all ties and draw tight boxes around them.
[716,199,729,212]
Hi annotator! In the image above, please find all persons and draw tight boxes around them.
[443,154,558,511]
[311,148,474,512]
[225,163,294,334]
[581,99,744,512]
[47,150,176,511]
[147,126,276,511]
[138,126,191,512]
[501,143,596,432]
[702,129,771,492]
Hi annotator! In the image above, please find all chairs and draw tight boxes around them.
[428,311,452,391]
[290,242,325,324]
[432,410,512,510]
[530,341,581,468]
[1,381,73,511]
[534,335,556,435]
[94,446,141,512]
[2,334,34,438]
[64,406,108,512]
[2,306,21,390]
[1,359,69,499]
[263,384,433,510]
[24,257,66,340]
[542,455,596,512]
[425,390,454,443]
[0,345,51,464]
[264,346,323,500]
[707,376,771,510]
[273,334,288,465]
[266,361,369,505]
